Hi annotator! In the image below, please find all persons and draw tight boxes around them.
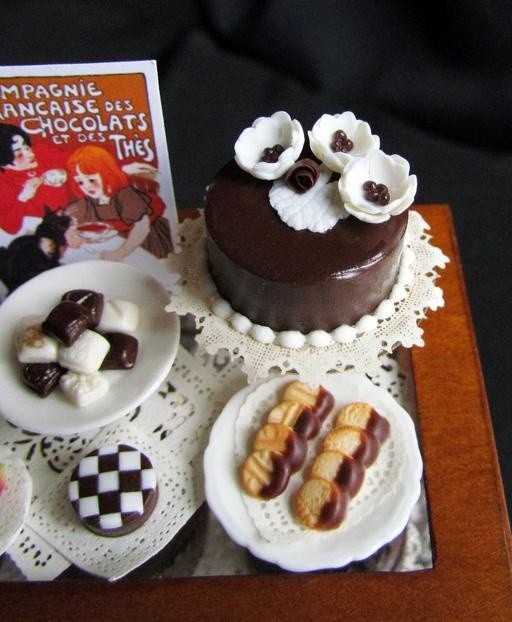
[1,121,79,249]
[60,145,173,264]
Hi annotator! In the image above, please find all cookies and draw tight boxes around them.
[242,377,390,529]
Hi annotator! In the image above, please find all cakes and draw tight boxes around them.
[195,109,417,346]
[12,289,140,408]
[66,444,159,537]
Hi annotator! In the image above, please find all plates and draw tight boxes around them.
[204,373,424,574]
[0,258,182,439]
